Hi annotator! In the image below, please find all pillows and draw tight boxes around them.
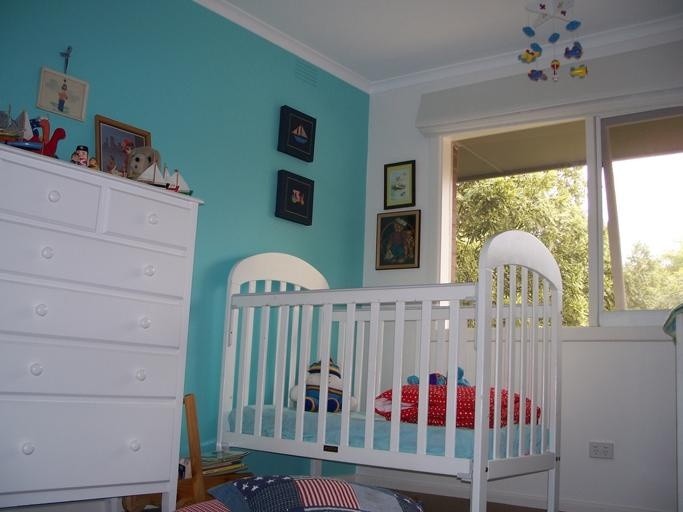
[206,473,426,511]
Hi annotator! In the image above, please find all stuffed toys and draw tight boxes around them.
[407,364,472,388]
[287,351,358,414]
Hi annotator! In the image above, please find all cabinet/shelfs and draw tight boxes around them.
[1,141,204,511]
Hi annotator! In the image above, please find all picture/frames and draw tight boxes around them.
[276,104,317,163]
[34,65,89,122]
[93,113,151,179]
[273,168,314,226]
[374,209,421,270]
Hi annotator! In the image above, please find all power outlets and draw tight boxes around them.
[586,441,615,460]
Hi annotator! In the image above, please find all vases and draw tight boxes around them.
[382,159,417,210]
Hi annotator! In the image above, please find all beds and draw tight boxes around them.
[208,227,566,512]
